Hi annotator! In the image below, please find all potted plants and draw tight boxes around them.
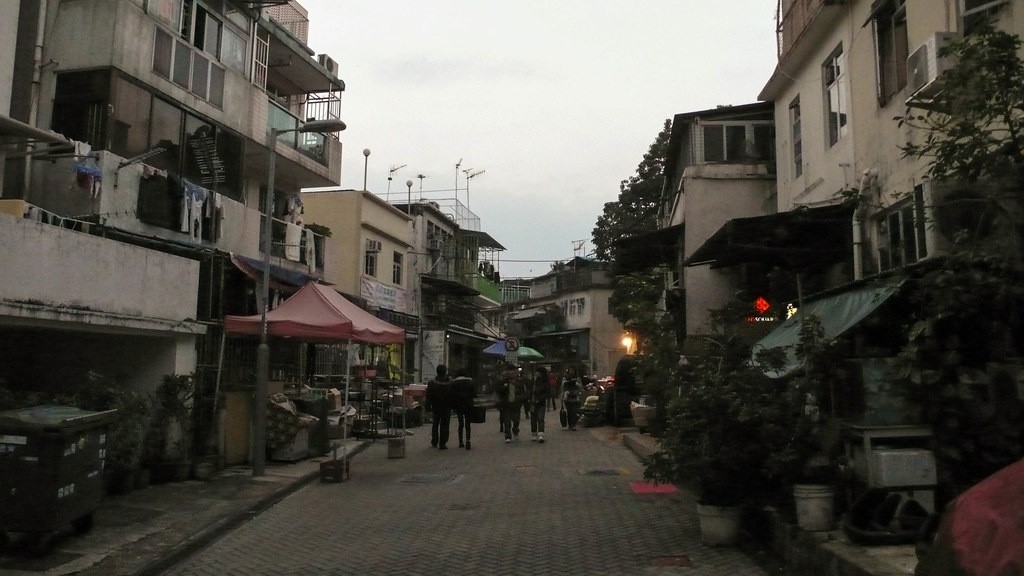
[69,371,225,489]
[646,294,841,547]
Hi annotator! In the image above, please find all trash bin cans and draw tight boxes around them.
[388,405,409,428]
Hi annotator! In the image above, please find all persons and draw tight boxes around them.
[426,365,476,450]
[492,358,597,442]
[914,368,1024,576]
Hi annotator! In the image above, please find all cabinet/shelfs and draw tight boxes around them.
[838,419,937,545]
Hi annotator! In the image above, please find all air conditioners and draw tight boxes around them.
[367,240,382,252]
[318,54,338,78]
[904,32,964,113]
[427,239,445,251]
[458,247,469,258]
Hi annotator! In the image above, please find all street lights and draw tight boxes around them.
[251,120,346,477]
[505,311,519,336]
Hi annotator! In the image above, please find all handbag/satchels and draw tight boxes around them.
[560,410,567,427]
[468,406,487,423]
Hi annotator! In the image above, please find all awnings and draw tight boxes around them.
[614,223,685,274]
[421,276,479,296]
[512,306,552,321]
[684,202,848,267]
[523,329,584,339]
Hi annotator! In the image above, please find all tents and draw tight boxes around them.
[210,281,407,481]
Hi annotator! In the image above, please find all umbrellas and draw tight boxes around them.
[483,342,508,357]
[517,347,543,360]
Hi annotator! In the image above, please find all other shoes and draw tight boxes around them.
[459,443,465,448]
[553,408,557,411]
[572,426,576,430]
[531,434,538,440]
[514,433,519,440]
[538,436,544,443]
[431,440,438,448]
[505,439,510,443]
[439,444,448,449]
[466,441,471,450]
[500,427,503,432]
[526,415,529,418]
[547,410,549,412]
